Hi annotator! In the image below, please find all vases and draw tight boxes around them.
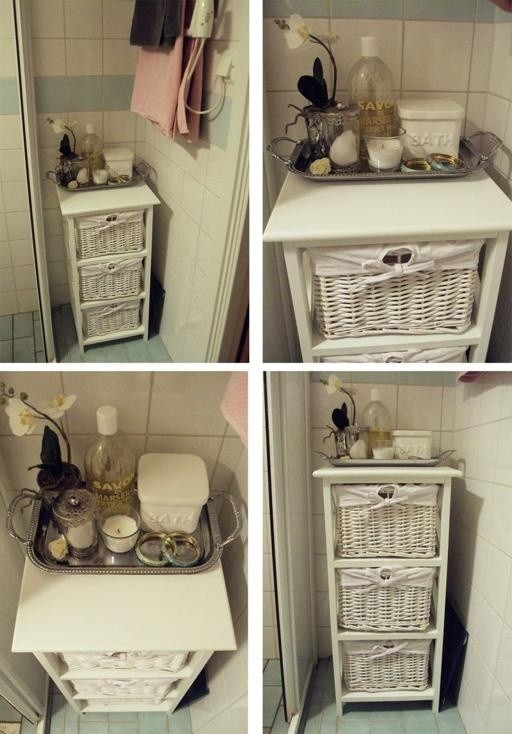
[54,149,94,185]
[328,422,371,460]
[29,458,82,509]
[298,101,357,160]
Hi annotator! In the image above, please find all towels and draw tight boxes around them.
[122,0,211,145]
[215,371,249,455]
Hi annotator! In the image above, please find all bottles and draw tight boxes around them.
[347,36,402,159]
[80,124,103,180]
[362,388,391,457]
[82,404,136,516]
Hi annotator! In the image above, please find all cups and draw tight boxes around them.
[72,155,90,186]
[372,439,395,459]
[97,504,142,556]
[343,425,370,458]
[91,162,109,185]
[363,124,407,171]
[319,107,361,173]
[51,487,99,557]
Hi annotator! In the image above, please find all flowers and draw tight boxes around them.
[0,380,81,499]
[45,110,85,160]
[312,371,359,433]
[271,11,344,135]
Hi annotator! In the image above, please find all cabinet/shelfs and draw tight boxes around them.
[310,457,466,716]
[52,166,163,355]
[6,546,239,717]
[259,153,509,364]
